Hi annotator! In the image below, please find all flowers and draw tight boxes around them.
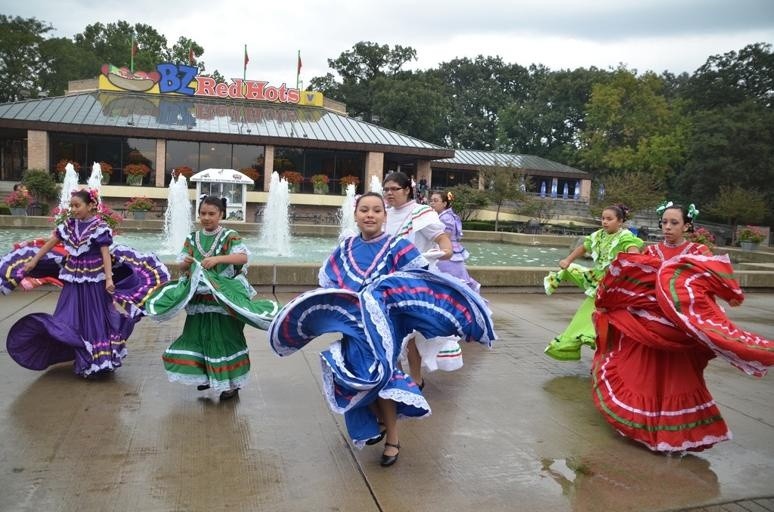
[48,203,123,238]
[734,224,768,244]
[447,192,454,202]
[3,183,33,208]
[123,196,155,212]
[85,187,99,200]
[688,227,716,249]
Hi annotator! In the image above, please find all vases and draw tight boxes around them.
[739,239,760,251]
[8,207,27,216]
[132,209,147,219]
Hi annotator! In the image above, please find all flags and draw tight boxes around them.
[297,56,302,75]
[189,48,193,64]
[244,51,249,69]
[131,40,136,56]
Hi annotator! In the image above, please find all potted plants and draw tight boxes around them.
[22,167,62,216]
[89,160,112,185]
[338,174,360,196]
[123,164,149,186]
[171,166,193,189]
[280,169,305,193]
[237,167,261,191]
[310,174,329,194]
[54,158,81,184]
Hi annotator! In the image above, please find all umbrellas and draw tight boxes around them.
[539,181,547,199]
[562,181,569,199]
[573,180,581,200]
[550,181,558,198]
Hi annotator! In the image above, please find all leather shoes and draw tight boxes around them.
[197,384,210,391]
[416,378,424,391]
[380,441,400,466]
[366,428,385,445]
[220,388,238,401]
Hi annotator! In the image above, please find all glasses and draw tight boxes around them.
[383,186,402,192]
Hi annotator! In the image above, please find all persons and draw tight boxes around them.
[315,197,430,468]
[376,172,455,393]
[406,174,427,204]
[22,189,116,378]
[558,203,645,351]
[178,194,251,403]
[428,191,483,295]
[589,200,742,456]
